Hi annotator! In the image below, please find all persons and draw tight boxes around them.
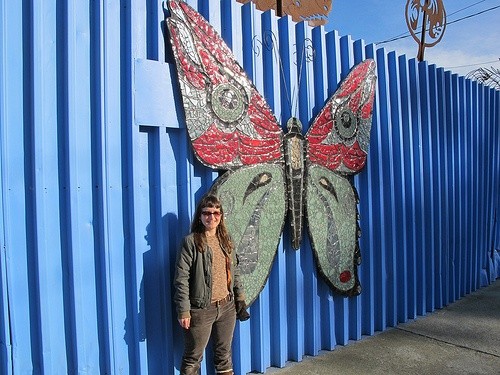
[175,197,250,375]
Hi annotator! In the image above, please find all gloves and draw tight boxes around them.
[235,293,251,322]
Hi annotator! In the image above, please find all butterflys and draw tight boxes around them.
[165,1,375,309]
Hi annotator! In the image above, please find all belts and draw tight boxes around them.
[210,293,230,307]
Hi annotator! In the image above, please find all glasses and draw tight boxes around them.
[202,211,223,216]
